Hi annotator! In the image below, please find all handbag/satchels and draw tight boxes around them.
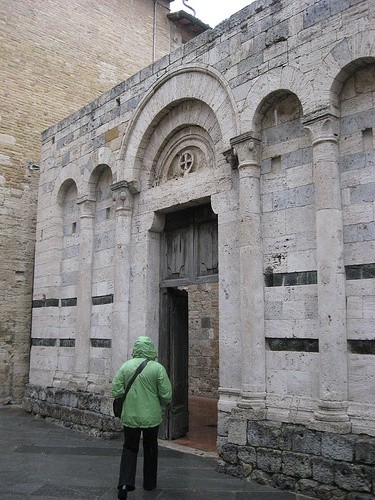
[112,395,125,418]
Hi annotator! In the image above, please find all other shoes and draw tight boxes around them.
[117,485,127,500]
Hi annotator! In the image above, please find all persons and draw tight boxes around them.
[111,336,173,500]
